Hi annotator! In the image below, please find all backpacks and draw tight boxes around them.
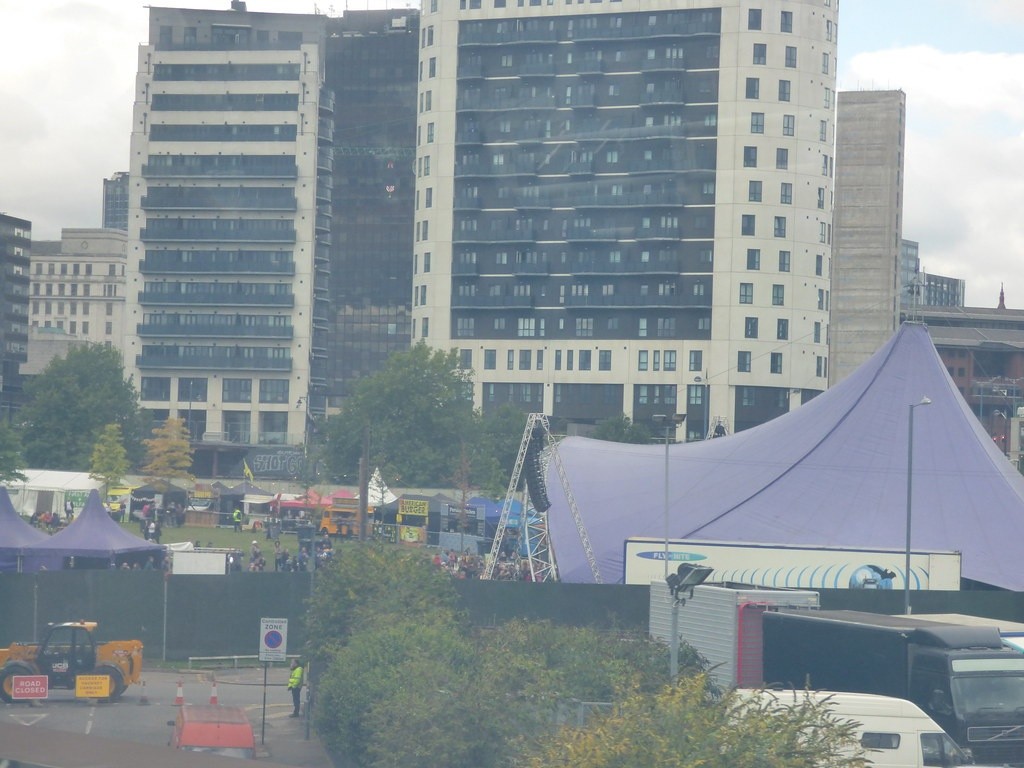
[236,511,241,519]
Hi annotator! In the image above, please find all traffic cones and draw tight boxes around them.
[172,681,191,706]
[208,682,223,705]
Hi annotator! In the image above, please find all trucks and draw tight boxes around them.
[0,620,143,702]
[760,609,1024,765]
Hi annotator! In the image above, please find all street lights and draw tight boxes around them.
[649,412,690,574]
[903,395,933,613]
[666,564,715,678]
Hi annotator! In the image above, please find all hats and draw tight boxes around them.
[252,540,257,544]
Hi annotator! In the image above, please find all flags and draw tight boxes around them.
[244,459,253,482]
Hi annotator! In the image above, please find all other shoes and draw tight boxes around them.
[289,713,299,717]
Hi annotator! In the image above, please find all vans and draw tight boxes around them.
[718,688,977,768]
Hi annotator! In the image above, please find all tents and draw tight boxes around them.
[544,324,1024,591]
[0,470,188,522]
[17,488,166,569]
[0,485,52,570]
[380,494,539,556]
[189,468,397,529]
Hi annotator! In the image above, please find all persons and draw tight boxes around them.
[288,659,304,717]
[434,549,532,581]
[234,507,242,531]
[194,541,201,550]
[120,556,169,571]
[65,497,74,524]
[334,515,353,542]
[29,511,60,533]
[274,534,335,572]
[141,502,183,544]
[205,542,213,547]
[106,502,112,517]
[119,500,126,522]
[39,562,47,570]
[248,541,266,571]
